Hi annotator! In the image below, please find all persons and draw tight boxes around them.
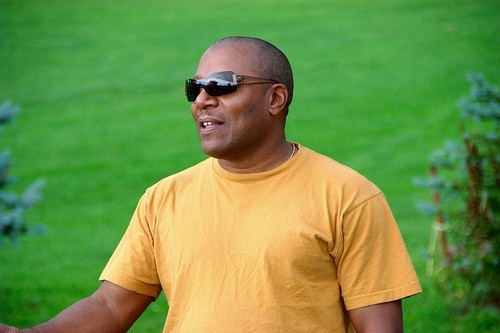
[0,35,423,333]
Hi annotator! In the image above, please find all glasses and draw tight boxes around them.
[184,70,279,102]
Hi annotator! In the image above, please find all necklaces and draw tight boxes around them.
[285,140,296,162]
